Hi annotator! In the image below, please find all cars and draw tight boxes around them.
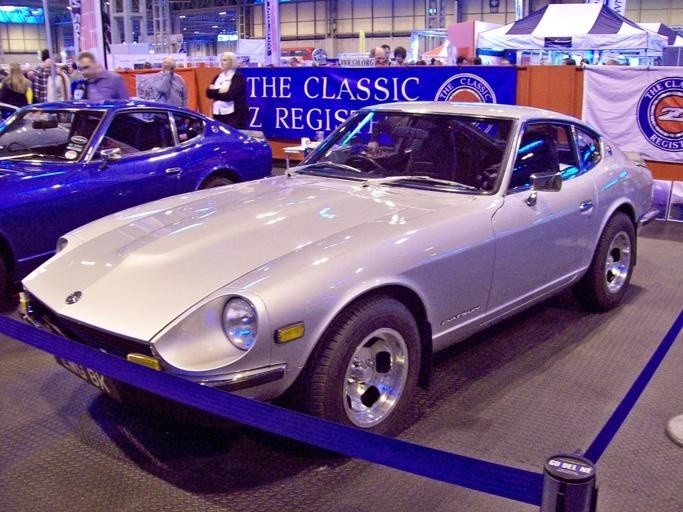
[0,100,272,316]
[16,101,660,437]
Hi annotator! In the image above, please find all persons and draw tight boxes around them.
[42,58,72,104]
[76,53,127,101]
[204,53,250,130]
[367,44,482,68]
[32,49,55,106]
[0,62,31,119]
[149,56,187,110]
[68,62,81,84]
